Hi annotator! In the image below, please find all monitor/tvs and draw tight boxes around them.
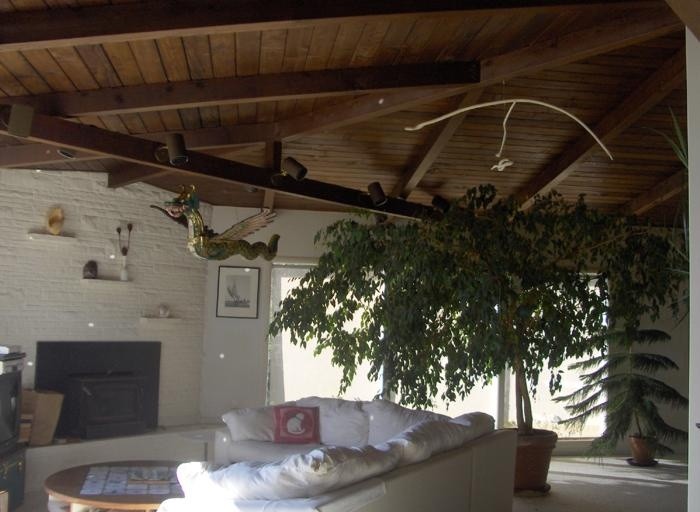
[1,372,22,456]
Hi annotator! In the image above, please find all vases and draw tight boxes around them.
[120,255,127,282]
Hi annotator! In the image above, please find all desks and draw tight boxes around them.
[44,457,187,512]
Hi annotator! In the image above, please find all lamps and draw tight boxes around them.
[271,156,307,186]
[429,192,450,216]
[154,131,189,168]
[354,181,388,209]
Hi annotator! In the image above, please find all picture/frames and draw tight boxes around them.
[216,263,260,321]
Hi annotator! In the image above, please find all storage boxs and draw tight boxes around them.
[20,387,66,446]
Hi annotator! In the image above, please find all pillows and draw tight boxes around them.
[273,404,321,445]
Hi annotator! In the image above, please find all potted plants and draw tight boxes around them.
[267,179,690,490]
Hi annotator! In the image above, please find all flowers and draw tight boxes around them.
[115,223,133,257]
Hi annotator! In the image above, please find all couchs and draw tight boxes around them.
[156,395,519,512]
